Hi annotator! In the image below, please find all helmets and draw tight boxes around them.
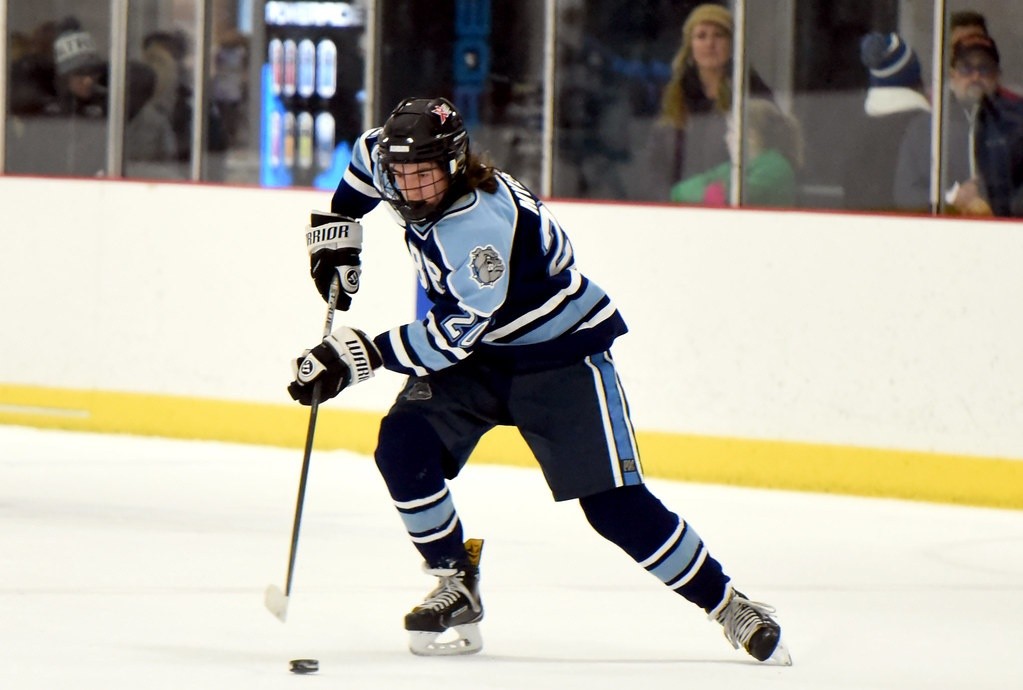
[377,97,470,226]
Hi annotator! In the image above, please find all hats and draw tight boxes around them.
[682,5,734,45]
[948,10,1000,70]
[861,32,921,88]
[51,16,101,77]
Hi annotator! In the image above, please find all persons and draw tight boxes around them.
[943,11,1023,217]
[836,30,977,213]
[668,96,804,208]
[287,96,794,666]
[7,15,251,184]
[638,4,780,201]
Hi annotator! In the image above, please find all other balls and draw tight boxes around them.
[289,658,320,674]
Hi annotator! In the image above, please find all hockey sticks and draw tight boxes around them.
[260,273,342,627]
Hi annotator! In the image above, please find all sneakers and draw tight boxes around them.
[404,538,484,656]
[706,582,793,666]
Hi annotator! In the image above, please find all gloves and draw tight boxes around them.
[288,324,383,406]
[306,211,363,311]
